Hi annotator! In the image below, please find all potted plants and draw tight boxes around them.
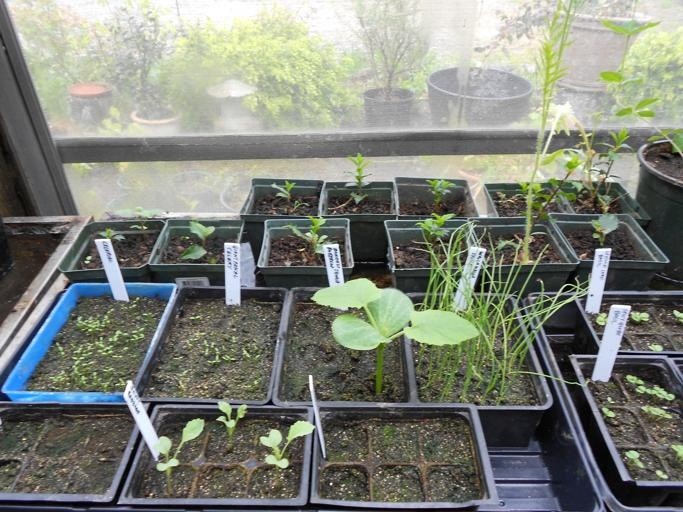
[25,0,185,136]
[544,0,655,128]
[349,2,430,128]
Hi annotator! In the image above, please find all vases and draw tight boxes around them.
[429,60,533,126]
